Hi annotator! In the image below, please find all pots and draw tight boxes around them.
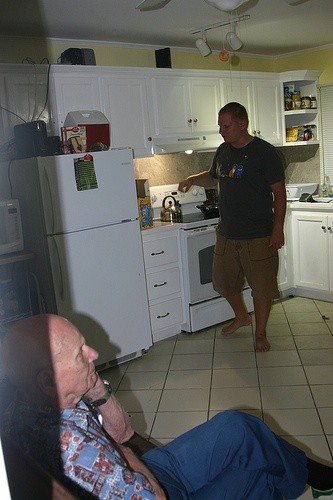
[196,199,220,217]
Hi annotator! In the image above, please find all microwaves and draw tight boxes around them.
[0,199,25,256]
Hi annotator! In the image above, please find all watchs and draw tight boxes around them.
[91,380,113,407]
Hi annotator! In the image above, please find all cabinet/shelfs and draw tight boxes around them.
[220,79,281,146]
[142,230,186,343]
[284,107,319,145]
[152,77,219,135]
[292,211,333,291]
[50,73,100,132]
[101,74,151,157]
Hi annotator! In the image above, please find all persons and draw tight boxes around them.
[3,312,333,500]
[178,103,287,352]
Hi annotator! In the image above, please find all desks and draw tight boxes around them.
[0,249,38,338]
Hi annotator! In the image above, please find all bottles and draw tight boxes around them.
[284,91,317,112]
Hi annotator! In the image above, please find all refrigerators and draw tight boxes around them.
[0,149,154,373]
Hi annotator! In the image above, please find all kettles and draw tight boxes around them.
[159,195,183,221]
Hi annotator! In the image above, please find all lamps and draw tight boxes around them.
[195,28,211,56]
[226,19,242,51]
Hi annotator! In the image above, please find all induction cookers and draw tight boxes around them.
[145,183,224,231]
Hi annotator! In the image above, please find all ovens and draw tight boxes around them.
[179,225,255,333]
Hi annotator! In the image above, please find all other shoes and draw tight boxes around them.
[311,485,333,498]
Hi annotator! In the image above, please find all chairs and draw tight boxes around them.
[6,399,157,499]
